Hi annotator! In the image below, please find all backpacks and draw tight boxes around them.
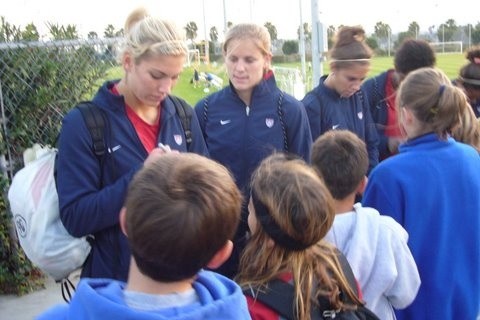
[7,105,117,283]
[239,247,380,320]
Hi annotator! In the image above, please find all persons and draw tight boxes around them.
[448,46,479,120]
[308,129,421,320]
[359,68,480,320]
[301,24,382,204]
[33,146,255,320]
[194,22,313,283]
[359,38,436,162]
[229,153,365,319]
[53,8,210,284]
